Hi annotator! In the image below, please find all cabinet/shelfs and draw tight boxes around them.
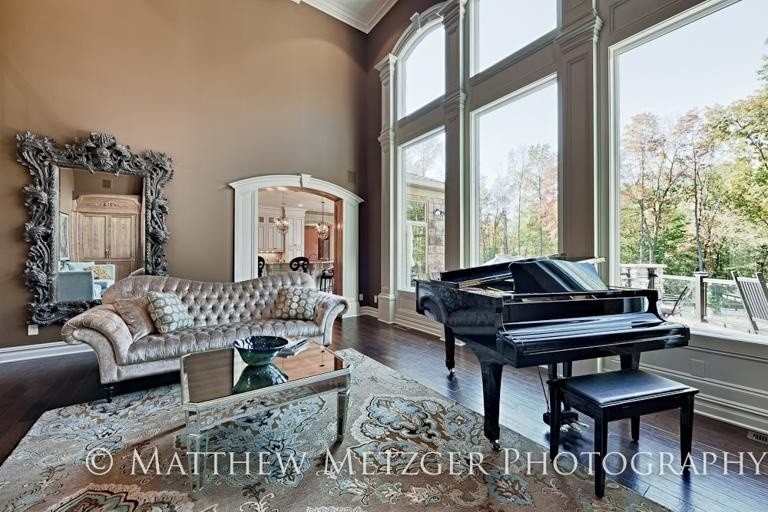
[72,195,141,261]
[95,262,138,283]
[258,206,284,254]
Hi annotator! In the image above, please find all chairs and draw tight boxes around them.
[320,270,334,292]
[288,257,309,273]
[258,256,264,277]
[60,261,115,301]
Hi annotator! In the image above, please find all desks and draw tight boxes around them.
[620,263,667,290]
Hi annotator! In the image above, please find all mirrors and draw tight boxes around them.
[14,132,175,330]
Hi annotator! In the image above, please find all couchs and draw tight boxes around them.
[60,270,348,403]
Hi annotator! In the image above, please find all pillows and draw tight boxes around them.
[84,266,111,280]
[112,295,156,344]
[271,286,318,321]
[66,263,91,270]
[146,290,194,335]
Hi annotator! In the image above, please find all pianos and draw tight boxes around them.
[414,252,691,454]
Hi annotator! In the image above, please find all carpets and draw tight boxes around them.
[0,348,677,512]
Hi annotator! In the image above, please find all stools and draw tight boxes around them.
[546,367,699,498]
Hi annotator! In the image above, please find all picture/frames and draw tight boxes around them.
[59,211,71,261]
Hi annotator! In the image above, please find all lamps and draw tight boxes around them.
[316,202,330,241]
[434,204,445,220]
[276,191,290,235]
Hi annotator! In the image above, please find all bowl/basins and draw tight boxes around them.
[231,336,289,367]
[231,363,288,394]
